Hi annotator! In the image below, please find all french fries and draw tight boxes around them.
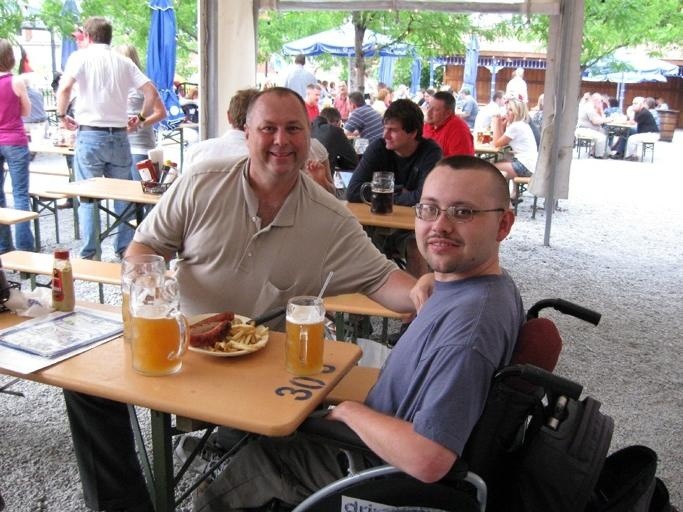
[204,324,269,351]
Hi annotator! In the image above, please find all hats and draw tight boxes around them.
[72,27,85,40]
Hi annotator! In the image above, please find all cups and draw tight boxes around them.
[122,256,186,376]
[476,126,493,145]
[361,172,396,215]
[286,295,326,378]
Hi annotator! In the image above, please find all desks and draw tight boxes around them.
[1,296,362,512]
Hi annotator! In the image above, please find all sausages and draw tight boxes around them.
[189,320,231,347]
[190,311,234,329]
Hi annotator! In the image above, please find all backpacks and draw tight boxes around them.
[498,396,678,512]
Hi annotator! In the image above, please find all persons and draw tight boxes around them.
[176,154,527,512]
[1,16,166,261]
[123,81,433,448]
[575,92,669,160]
[284,54,545,346]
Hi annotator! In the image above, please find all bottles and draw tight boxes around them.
[48,247,77,314]
[352,137,369,154]
[132,148,179,183]
[39,87,57,110]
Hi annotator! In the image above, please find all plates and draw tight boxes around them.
[183,313,272,358]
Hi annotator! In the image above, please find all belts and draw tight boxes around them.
[80,125,127,132]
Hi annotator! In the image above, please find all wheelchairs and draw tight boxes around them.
[288,296,671,512]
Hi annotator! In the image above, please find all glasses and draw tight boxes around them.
[414,202,504,225]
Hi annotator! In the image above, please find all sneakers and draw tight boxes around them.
[173,435,235,480]
